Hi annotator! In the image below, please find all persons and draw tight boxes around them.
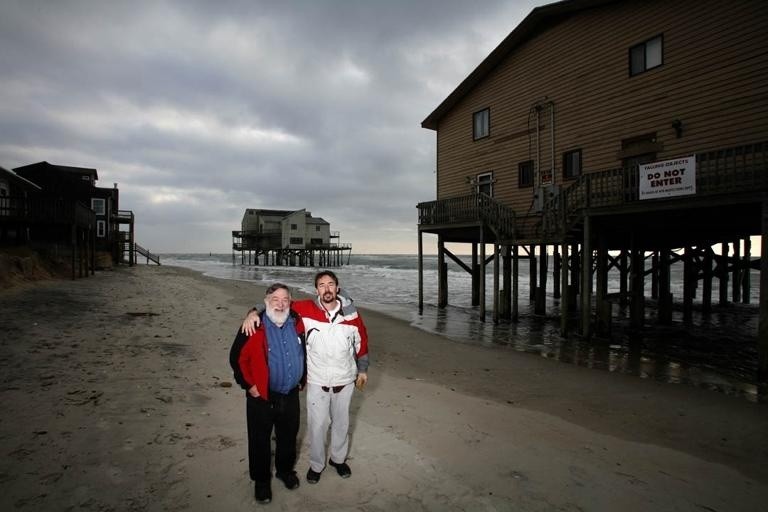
[240,271,369,485]
[229,283,307,504]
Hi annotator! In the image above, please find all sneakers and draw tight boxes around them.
[305,462,328,485]
[328,455,355,480]
[275,469,302,491]
[252,477,275,505]
